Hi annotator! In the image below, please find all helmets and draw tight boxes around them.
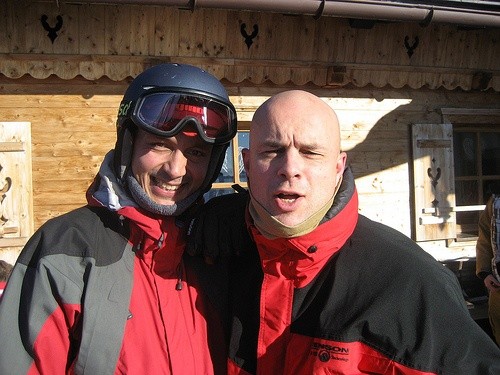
[115,62,230,173]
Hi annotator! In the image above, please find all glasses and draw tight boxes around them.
[130,86,238,143]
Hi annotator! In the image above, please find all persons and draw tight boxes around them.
[475,189,500,345]
[185,90,500,375]
[0,62,239,375]
